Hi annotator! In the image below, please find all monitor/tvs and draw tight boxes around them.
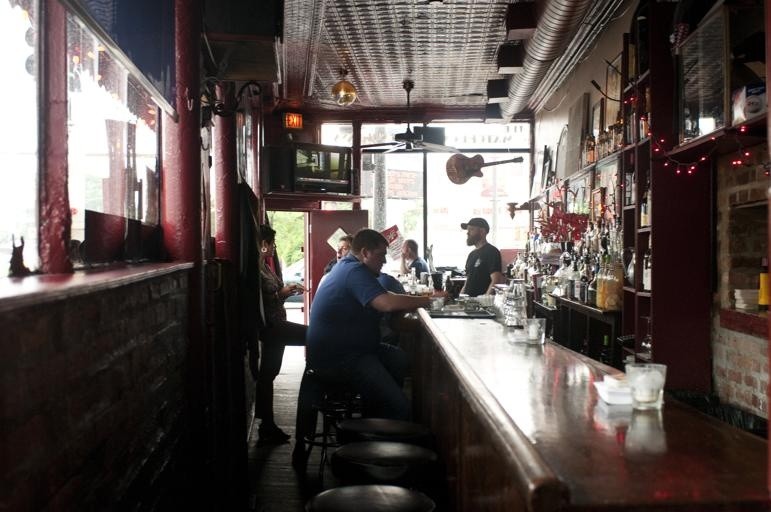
[292,142,351,192]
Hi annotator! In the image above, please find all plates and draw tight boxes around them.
[591,380,664,406]
[442,304,465,312]
[590,403,631,426]
[733,288,760,311]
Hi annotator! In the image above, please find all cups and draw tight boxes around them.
[453,293,497,313]
[423,296,444,313]
[624,361,668,412]
[622,408,669,463]
[431,273,443,291]
[444,270,452,279]
[419,271,428,285]
[504,280,528,327]
[522,317,548,345]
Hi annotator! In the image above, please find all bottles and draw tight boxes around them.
[511,233,556,308]
[640,319,651,355]
[445,275,451,293]
[756,257,769,312]
[409,266,417,295]
[622,151,651,231]
[578,337,588,355]
[683,102,697,145]
[641,232,652,294]
[581,111,649,166]
[557,206,621,315]
[598,334,611,367]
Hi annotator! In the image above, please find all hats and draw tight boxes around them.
[461,218,489,229]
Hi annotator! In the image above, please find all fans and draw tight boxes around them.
[351,80,461,155]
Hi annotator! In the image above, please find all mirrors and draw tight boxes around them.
[678,5,730,146]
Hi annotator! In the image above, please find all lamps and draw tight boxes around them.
[331,70,358,107]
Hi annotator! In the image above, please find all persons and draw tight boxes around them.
[254,225,310,444]
[304,229,435,421]
[292,270,410,470]
[337,235,353,263]
[459,218,503,297]
[399,239,429,280]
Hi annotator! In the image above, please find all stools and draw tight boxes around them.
[298,376,437,511]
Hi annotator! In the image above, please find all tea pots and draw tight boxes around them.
[618,245,635,289]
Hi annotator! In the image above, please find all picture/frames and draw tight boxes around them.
[532,122,568,193]
[603,51,625,129]
[562,88,588,177]
[588,97,603,135]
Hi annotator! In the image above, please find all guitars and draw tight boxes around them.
[446,154,523,185]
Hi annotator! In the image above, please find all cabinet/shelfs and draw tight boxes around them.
[653,68,771,170]
[521,145,716,386]
[622,18,672,143]
